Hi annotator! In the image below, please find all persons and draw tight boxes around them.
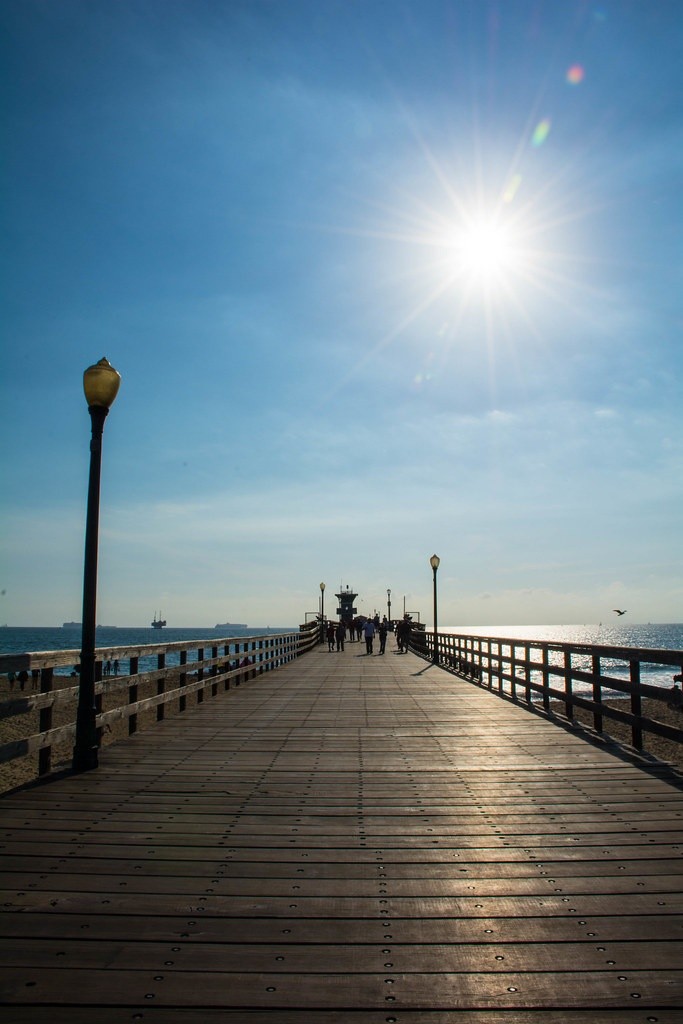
[17,671,28,691]
[31,669,40,691]
[326,613,413,655]
[210,656,251,677]
[103,660,120,676]
[8,672,17,690]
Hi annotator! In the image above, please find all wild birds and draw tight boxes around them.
[613,609,627,616]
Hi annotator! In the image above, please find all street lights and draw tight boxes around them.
[430,554,441,663]
[386,589,392,629]
[73,357,123,773]
[320,582,326,643]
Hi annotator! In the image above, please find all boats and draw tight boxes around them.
[215,622,248,629]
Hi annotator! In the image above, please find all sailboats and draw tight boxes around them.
[151,610,167,629]
